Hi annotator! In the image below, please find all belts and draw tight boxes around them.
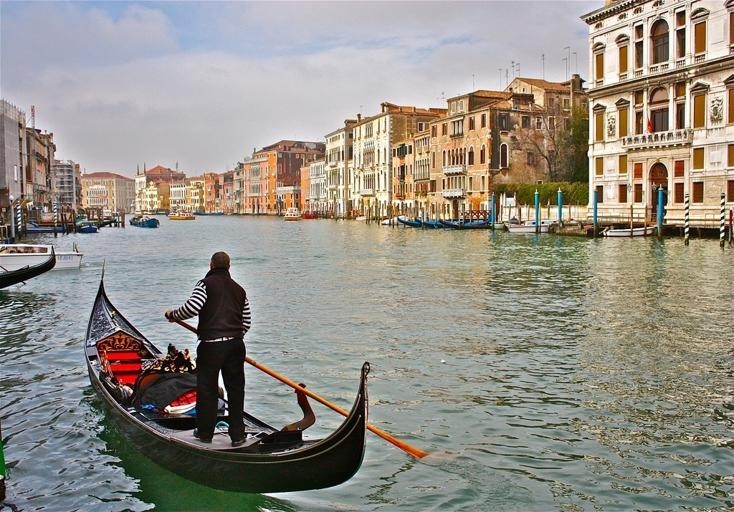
[204,336,235,343]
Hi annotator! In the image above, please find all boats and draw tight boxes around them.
[300,210,318,220]
[353,215,374,222]
[0,241,83,272]
[283,207,300,222]
[75,220,98,233]
[600,224,660,239]
[380,212,564,234]
[84,261,373,494]
[0,245,59,290]
[127,213,160,229]
[98,209,113,221]
[648,223,675,236]
[167,208,194,221]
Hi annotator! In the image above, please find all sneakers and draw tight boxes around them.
[193,428,214,443]
[230,434,248,447]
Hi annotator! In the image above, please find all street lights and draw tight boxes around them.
[8,192,15,240]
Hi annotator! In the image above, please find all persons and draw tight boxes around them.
[164,251,251,446]
